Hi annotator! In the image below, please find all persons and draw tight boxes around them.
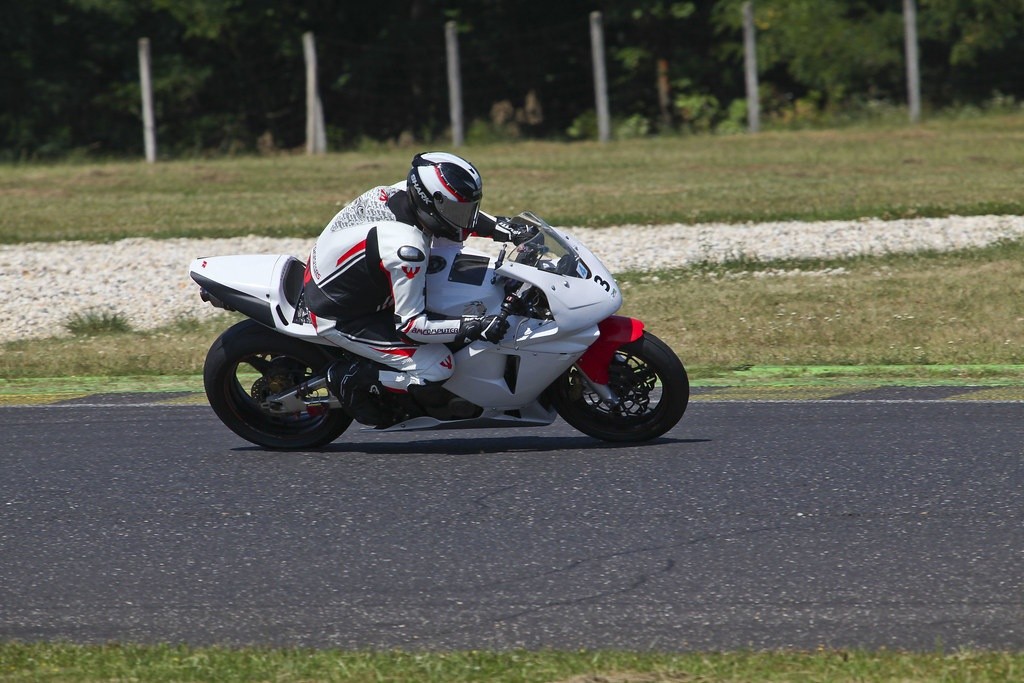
[304,151,545,426]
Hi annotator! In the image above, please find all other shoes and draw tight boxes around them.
[320,358,390,425]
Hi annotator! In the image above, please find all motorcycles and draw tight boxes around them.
[188,210,690,451]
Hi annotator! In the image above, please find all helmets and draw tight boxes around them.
[406,150,482,242]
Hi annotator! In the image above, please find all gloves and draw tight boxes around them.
[451,314,511,349]
[493,215,540,247]
